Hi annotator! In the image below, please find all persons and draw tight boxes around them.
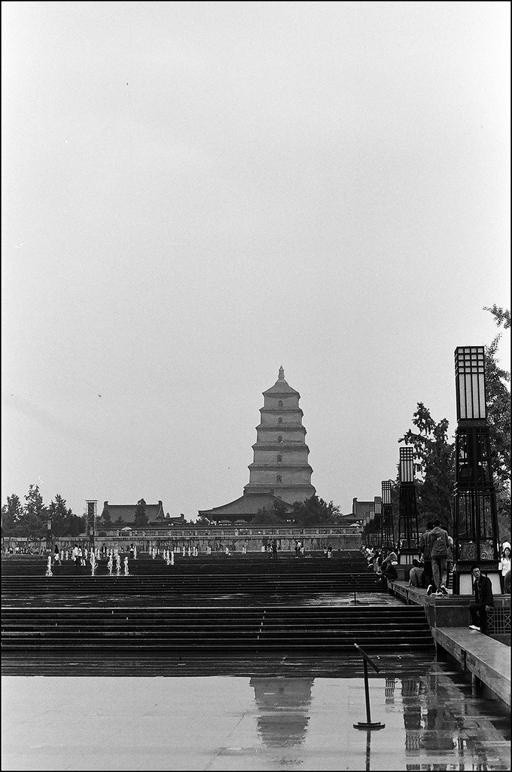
[322,542,336,559]
[294,540,307,560]
[469,566,494,636]
[359,519,462,598]
[487,535,511,595]
[183,539,247,560]
[0,530,135,567]
[261,537,278,560]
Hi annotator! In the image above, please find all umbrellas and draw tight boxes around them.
[122,526,132,531]
[350,523,360,527]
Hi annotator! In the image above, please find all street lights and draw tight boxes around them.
[445,346,504,595]
[362,481,396,548]
[399,447,422,566]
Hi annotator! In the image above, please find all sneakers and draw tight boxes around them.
[469,624,481,631]
[426,584,448,596]
